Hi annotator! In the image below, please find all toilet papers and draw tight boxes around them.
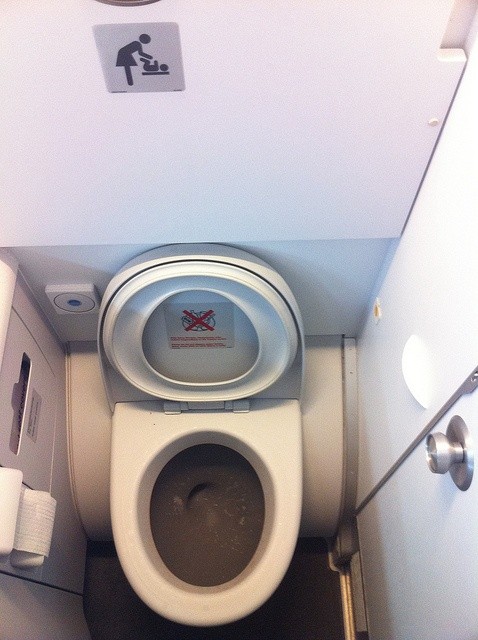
[13,486,59,559]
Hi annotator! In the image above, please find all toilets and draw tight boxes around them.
[96,243,305,629]
